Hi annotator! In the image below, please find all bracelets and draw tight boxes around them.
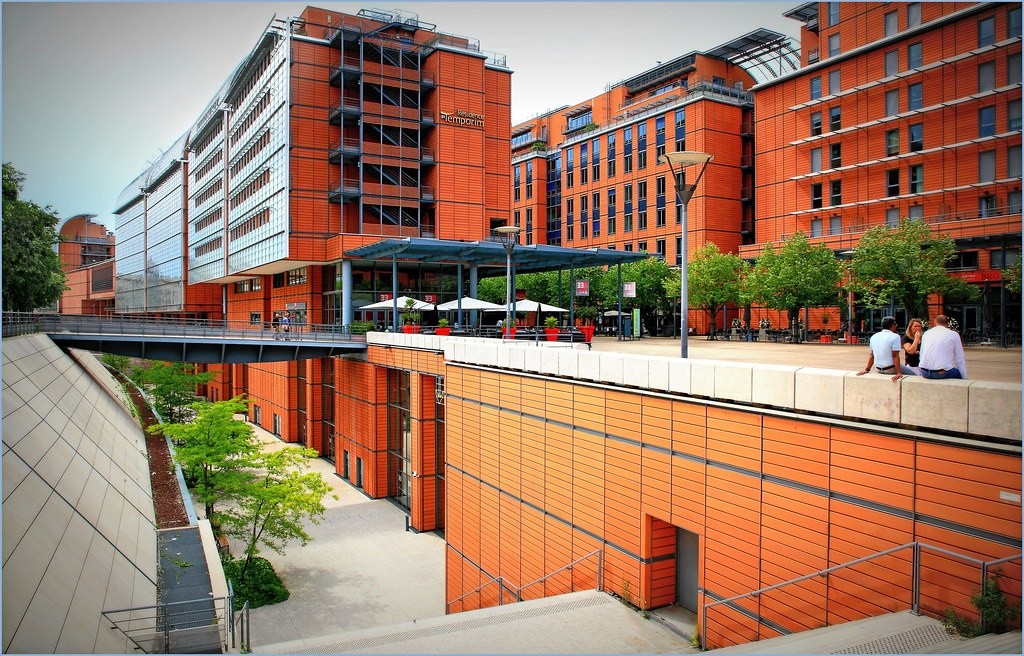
[865,368,870,371]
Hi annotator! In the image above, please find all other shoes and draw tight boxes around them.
[276,338,279,340]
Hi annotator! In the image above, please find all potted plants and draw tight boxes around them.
[501,317,517,339]
[575,305,597,342]
[544,316,560,341]
[401,298,451,337]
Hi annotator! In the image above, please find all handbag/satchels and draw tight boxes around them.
[282,325,289,330]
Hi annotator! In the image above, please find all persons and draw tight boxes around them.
[857,314,968,384]
[271,311,292,340]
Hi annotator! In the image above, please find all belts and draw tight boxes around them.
[921,367,944,373]
[876,365,895,371]
